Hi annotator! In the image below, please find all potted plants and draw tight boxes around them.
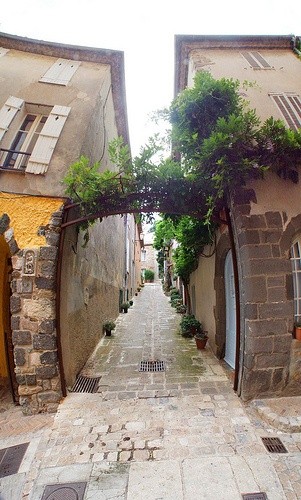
[120,303,130,313]
[167,285,209,350]
[135,284,144,296]
[129,301,133,306]
[103,320,116,336]
